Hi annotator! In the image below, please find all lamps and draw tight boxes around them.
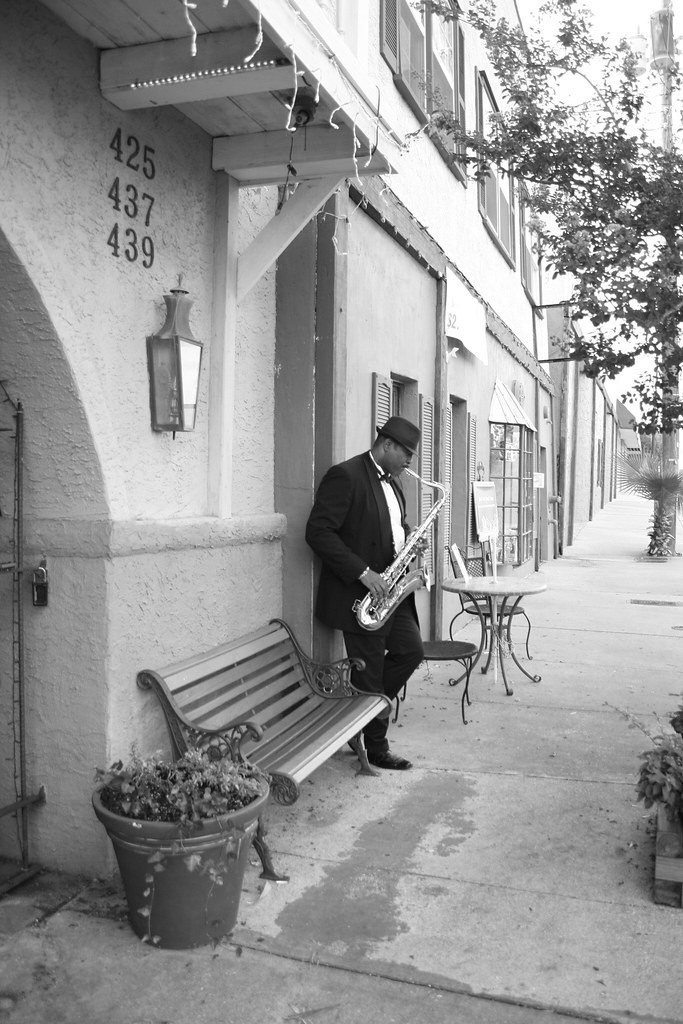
[146,272,205,440]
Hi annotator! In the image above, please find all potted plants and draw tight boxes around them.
[86,747,273,949]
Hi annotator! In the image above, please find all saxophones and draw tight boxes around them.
[349,467,449,633]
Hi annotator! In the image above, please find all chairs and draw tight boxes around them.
[391,640,478,726]
[445,541,533,663]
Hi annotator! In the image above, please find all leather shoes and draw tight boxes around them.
[349,736,413,770]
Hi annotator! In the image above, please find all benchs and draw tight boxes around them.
[134,619,394,882]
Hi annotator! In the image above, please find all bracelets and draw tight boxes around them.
[357,566,370,580]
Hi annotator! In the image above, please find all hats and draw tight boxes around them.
[376,416,422,457]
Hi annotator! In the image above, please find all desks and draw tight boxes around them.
[439,576,548,697]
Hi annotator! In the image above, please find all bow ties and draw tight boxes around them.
[379,475,393,482]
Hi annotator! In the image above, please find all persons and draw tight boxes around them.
[304,417,430,770]
